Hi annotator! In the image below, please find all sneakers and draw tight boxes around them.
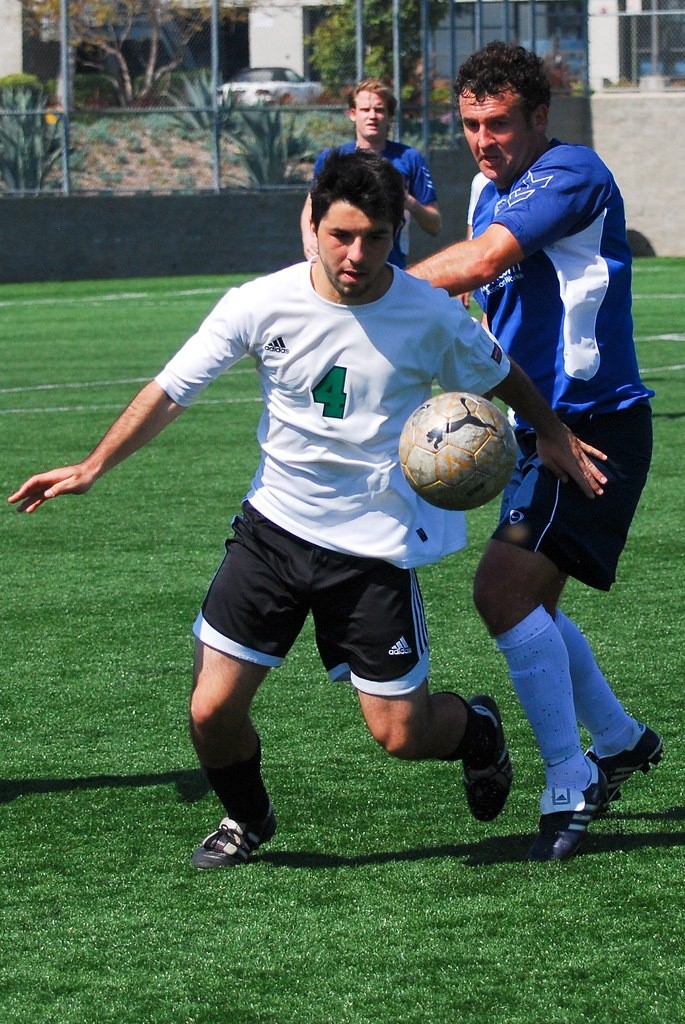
[582,725,663,814]
[194,804,276,871]
[527,757,611,864]
[462,693,512,821]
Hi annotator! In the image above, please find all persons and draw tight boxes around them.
[407,41,663,858]
[8,148,609,868]
[457,172,491,307]
[302,78,441,271]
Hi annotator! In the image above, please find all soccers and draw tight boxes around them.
[397,390,520,512]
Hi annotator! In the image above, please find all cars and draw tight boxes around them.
[222,67,324,109]
